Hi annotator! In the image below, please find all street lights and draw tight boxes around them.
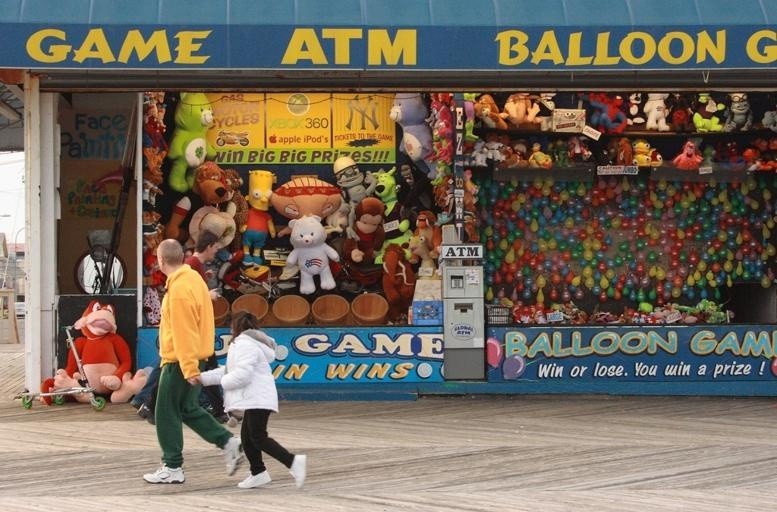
[1,214,12,219]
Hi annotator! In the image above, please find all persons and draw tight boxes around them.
[140,236,246,485]
[197,310,308,491]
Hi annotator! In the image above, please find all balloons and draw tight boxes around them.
[477,177,777,302]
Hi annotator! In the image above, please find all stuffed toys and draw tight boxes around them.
[463,90,777,172]
[140,91,459,325]
[42,300,149,407]
[464,90,777,172]
[140,91,460,326]
[463,213,480,242]
[463,192,480,208]
[466,169,480,194]
[493,302,737,325]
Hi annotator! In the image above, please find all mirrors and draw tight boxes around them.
[73,249,128,295]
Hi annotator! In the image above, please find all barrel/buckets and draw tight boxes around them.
[351,293,389,325]
[212,294,228,326]
[231,293,268,324]
[272,294,310,325]
[312,295,350,325]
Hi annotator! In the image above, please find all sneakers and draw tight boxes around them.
[144,464,186,485]
[289,454,307,487]
[237,469,273,489]
[223,435,245,476]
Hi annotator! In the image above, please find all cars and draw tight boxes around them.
[14,294,24,316]
